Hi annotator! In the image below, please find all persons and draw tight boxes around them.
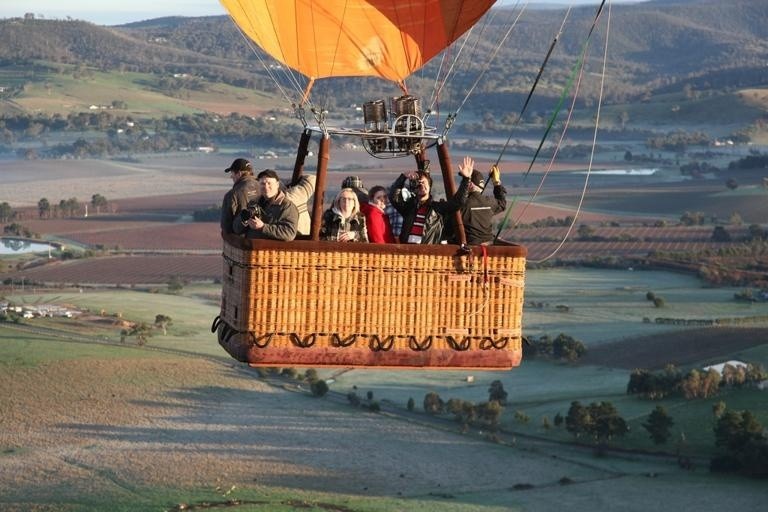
[221,156,506,244]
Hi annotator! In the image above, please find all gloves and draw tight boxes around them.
[490,165,500,184]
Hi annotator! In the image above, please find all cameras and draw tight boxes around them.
[241,204,261,220]
[410,179,419,192]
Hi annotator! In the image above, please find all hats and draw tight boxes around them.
[224,158,253,174]
[258,169,279,180]
[458,169,484,189]
